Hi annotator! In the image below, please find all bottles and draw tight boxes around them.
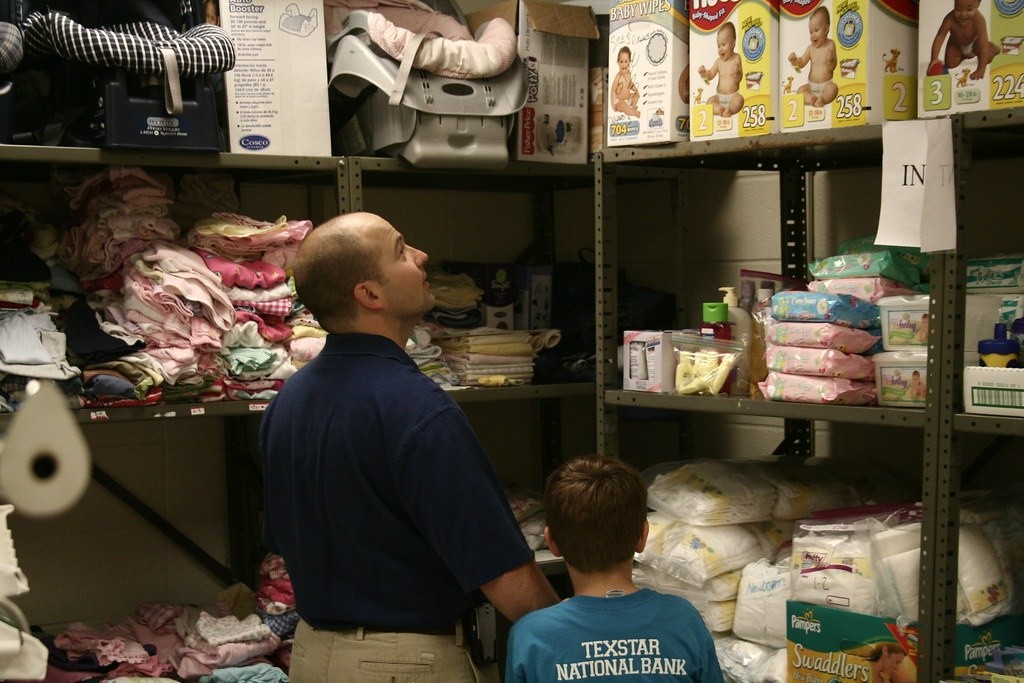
[977,324,1020,369]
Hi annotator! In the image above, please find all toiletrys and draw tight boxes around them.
[700,302,730,395]
[752,289,773,398]
[629,340,661,382]
[718,287,752,397]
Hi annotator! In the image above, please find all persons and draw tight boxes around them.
[910,371,926,399]
[610,46,641,120]
[927,0,1001,80]
[697,22,744,118]
[789,7,839,107]
[870,642,907,683]
[919,313,929,341]
[260,211,560,683]
[504,453,726,683]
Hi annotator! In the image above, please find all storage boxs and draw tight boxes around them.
[779,0,919,132]
[689,0,780,142]
[621,329,676,393]
[202,0,332,157]
[917,0,1023,117]
[607,0,689,147]
[465,0,600,164]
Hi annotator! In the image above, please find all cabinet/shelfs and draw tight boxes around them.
[592,107,945,683]
[0,145,351,584]
[929,106,1024,683]
[346,142,588,683]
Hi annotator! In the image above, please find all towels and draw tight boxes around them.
[405,325,534,386]
